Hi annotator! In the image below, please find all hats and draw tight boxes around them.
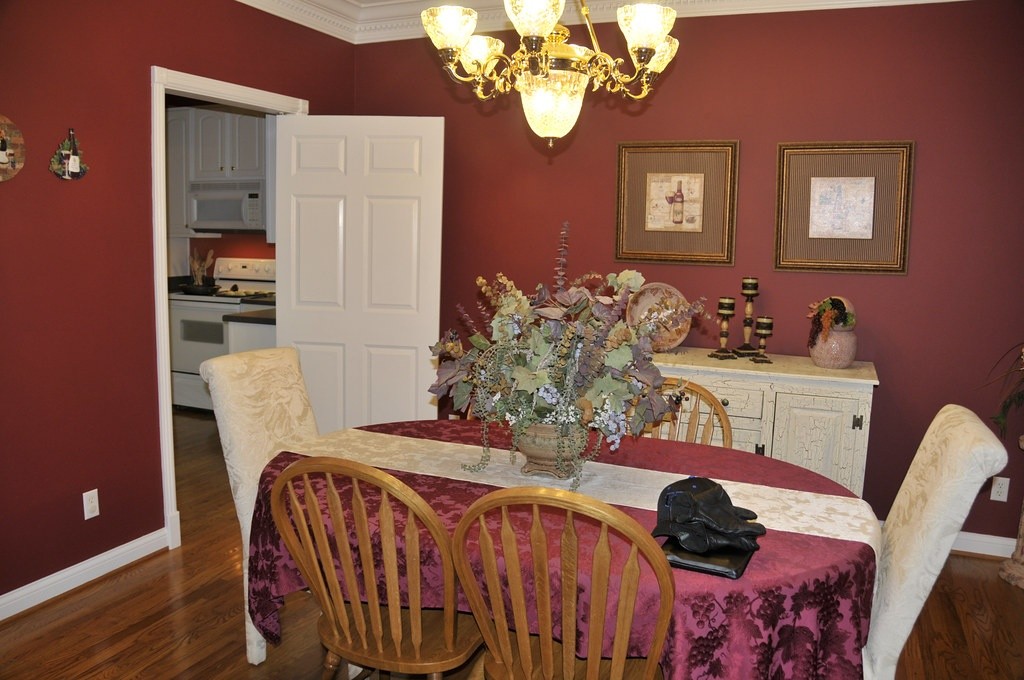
[650,477,732,539]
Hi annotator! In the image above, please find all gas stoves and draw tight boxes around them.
[168,257,277,303]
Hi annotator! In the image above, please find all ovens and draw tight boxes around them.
[168,301,240,411]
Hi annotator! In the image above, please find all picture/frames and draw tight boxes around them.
[772,141,917,275]
[616,138,736,268]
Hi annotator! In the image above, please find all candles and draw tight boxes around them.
[741,274,759,297]
[754,314,774,336]
[716,296,735,315]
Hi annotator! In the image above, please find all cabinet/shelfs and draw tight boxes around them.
[644,368,872,499]
[159,103,268,237]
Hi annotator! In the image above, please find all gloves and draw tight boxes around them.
[669,521,760,552]
[673,483,766,536]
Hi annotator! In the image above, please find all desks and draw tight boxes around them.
[247,421,882,680]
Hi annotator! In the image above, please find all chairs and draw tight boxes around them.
[271,456,485,680]
[200,346,321,665]
[862,402,1008,680]
[453,482,676,680]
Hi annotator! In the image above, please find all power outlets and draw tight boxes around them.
[990,475,1011,503]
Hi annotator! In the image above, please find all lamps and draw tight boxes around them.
[420,0,680,151]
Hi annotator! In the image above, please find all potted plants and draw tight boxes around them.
[426,222,724,492]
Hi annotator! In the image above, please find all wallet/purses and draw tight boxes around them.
[666,545,755,579]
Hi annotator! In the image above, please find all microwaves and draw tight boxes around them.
[186,189,263,231]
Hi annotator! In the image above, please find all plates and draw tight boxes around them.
[625,282,692,351]
